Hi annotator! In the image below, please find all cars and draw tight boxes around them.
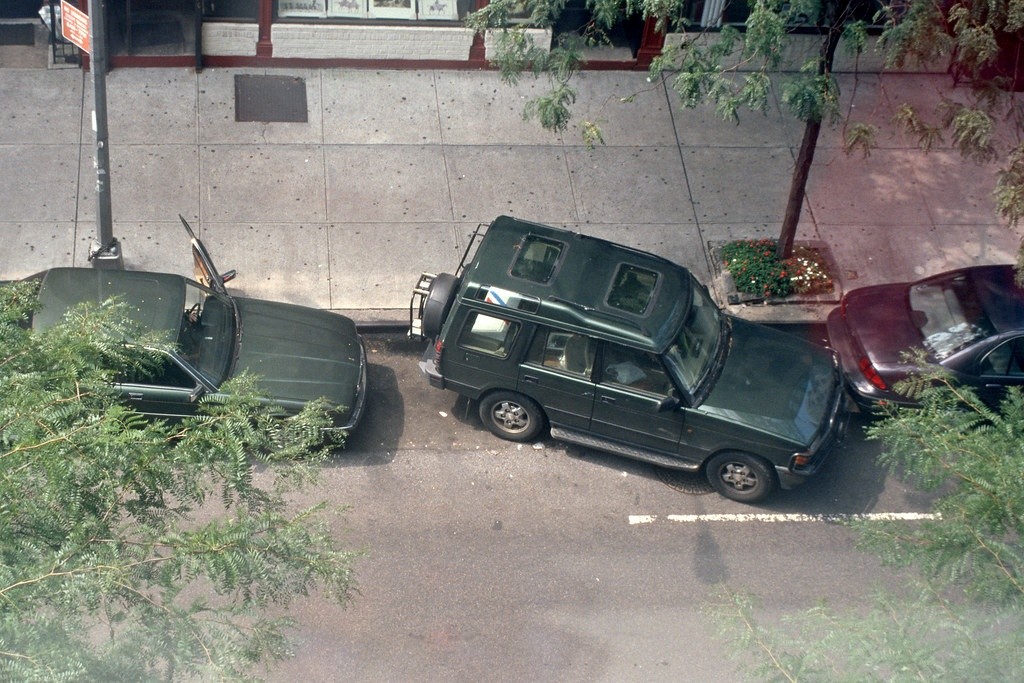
[0,214,365,460]
[824,265,1024,439]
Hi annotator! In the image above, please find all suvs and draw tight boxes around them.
[407,215,850,502]
